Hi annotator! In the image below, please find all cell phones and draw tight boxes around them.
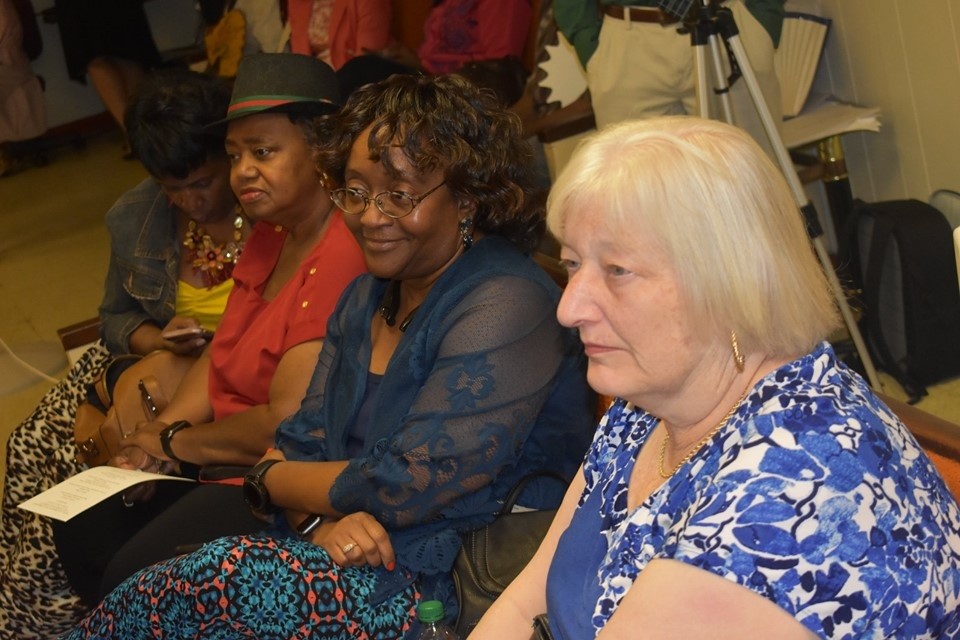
[161,326,214,343]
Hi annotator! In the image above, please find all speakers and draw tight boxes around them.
[844,199,960,386]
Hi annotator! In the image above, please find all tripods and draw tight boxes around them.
[673,3,882,395]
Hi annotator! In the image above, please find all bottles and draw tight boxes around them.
[413,600,459,640]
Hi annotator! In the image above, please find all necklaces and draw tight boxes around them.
[180,201,245,293]
[659,382,746,478]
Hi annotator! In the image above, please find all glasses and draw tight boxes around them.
[326,180,444,218]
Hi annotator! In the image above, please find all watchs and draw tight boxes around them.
[159,420,194,459]
[296,513,328,542]
[242,459,285,515]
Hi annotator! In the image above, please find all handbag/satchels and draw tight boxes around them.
[451,471,569,640]
[72,347,195,468]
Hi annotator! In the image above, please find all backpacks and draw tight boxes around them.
[833,197,960,400]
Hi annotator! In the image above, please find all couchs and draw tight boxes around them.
[22,0,200,134]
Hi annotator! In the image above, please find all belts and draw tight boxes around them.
[601,0,678,28]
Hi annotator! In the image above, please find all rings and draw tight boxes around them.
[124,430,133,438]
[341,542,359,555]
[121,494,134,507]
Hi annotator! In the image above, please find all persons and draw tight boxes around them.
[459,115,960,640]
[67,66,595,640]
[551,0,783,166]
[48,53,371,611]
[55,0,163,160]
[0,0,46,176]
[180,0,591,192]
[0,67,252,639]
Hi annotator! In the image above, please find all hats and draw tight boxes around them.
[202,51,345,133]
